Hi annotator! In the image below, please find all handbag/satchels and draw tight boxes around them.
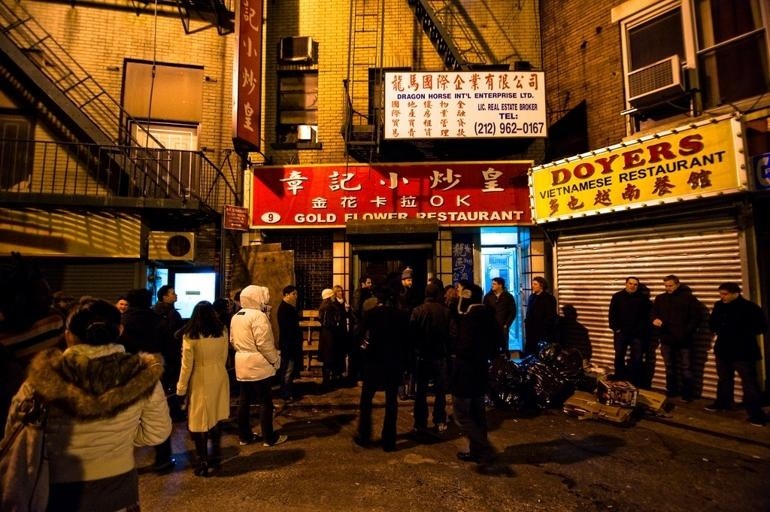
[0,400,50,512]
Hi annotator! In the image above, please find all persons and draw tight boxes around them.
[51,285,184,476]
[276,285,304,399]
[353,278,514,476]
[609,277,649,389]
[0,299,173,511]
[704,282,766,427]
[649,275,701,400]
[315,285,350,390]
[523,277,556,355]
[347,268,413,368]
[177,285,287,476]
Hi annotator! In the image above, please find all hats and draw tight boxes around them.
[322,289,334,299]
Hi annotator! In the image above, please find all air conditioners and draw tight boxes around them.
[146,228,198,262]
[278,33,315,64]
[624,53,688,106]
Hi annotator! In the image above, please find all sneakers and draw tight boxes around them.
[240,432,258,446]
[263,435,287,446]
